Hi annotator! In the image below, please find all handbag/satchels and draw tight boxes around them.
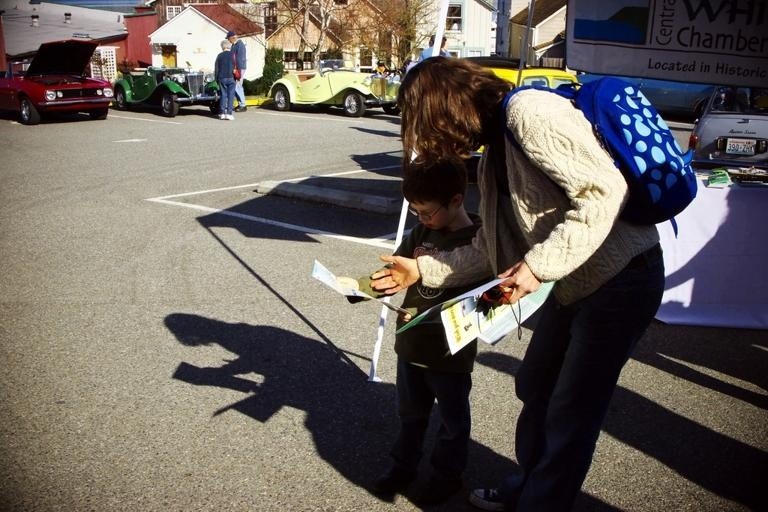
[233,69,241,80]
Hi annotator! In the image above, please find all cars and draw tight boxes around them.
[266,59,400,118]
[0,39,223,126]
[687,83,768,170]
[458,57,583,156]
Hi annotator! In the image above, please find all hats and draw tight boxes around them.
[225,31,235,39]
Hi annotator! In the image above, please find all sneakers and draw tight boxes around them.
[468,487,509,512]
[366,470,465,507]
[220,105,247,120]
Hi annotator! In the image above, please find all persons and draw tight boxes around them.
[215,32,247,120]
[418,35,451,64]
[338,149,492,509]
[370,58,664,511]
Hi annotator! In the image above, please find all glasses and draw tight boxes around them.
[408,204,443,222]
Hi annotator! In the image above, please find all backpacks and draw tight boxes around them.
[500,75,698,240]
